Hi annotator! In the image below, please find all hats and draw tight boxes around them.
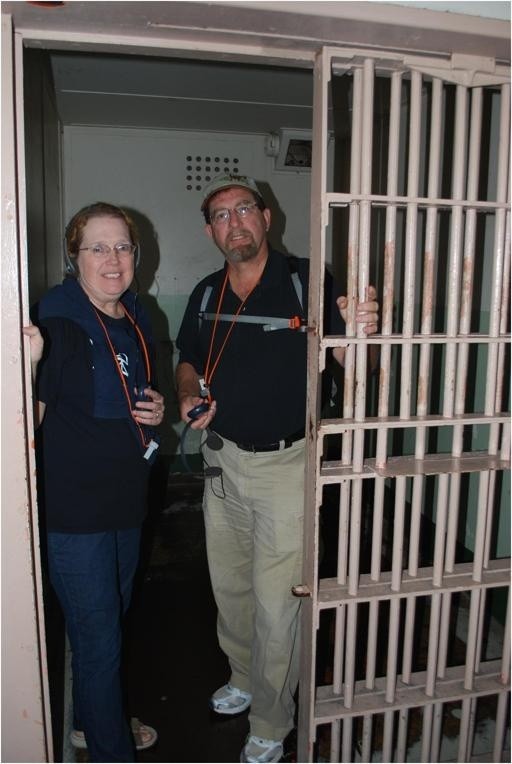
[200,176,262,211]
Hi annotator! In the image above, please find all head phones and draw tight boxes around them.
[181,418,224,481]
[61,203,142,278]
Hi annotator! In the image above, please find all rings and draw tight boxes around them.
[156,412,159,419]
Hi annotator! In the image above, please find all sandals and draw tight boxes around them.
[70,718,157,750]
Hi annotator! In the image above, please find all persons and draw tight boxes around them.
[20,200,182,763]
[175,172,380,762]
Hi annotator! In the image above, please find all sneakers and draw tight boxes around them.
[210,684,251,715]
[239,734,284,763]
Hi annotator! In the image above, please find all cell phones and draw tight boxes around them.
[137,383,152,412]
[186,402,211,418]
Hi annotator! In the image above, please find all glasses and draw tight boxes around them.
[210,203,260,225]
[80,243,137,259]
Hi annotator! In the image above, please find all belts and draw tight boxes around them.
[226,432,305,455]
